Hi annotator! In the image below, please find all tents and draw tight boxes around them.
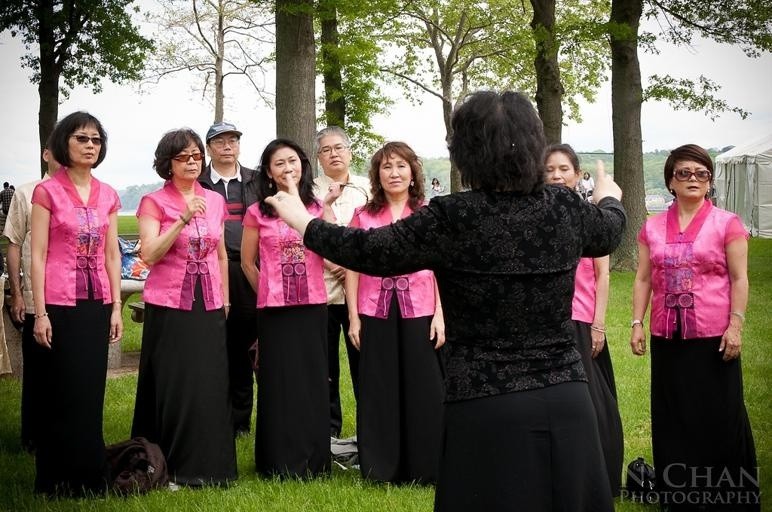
[712,134,772,241]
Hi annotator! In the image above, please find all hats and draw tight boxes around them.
[205,122,243,143]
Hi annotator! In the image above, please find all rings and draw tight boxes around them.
[591,345,597,349]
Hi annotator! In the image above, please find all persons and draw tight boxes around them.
[628,143,762,512]
[238,138,347,485]
[431,177,445,197]
[134,127,241,491]
[545,143,624,499]
[579,170,596,200]
[0,134,68,457]
[340,137,447,488]
[309,125,373,442]
[197,120,262,442]
[29,111,124,502]
[0,181,16,216]
[263,88,624,511]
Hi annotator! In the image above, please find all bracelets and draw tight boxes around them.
[730,308,747,324]
[34,312,50,319]
[631,318,643,328]
[590,326,609,332]
[112,299,124,305]
[177,215,190,228]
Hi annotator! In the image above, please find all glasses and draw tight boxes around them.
[173,152,205,163]
[70,134,104,145]
[673,168,711,183]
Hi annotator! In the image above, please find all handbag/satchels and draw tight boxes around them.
[329,433,359,471]
[625,456,658,506]
[116,236,152,281]
